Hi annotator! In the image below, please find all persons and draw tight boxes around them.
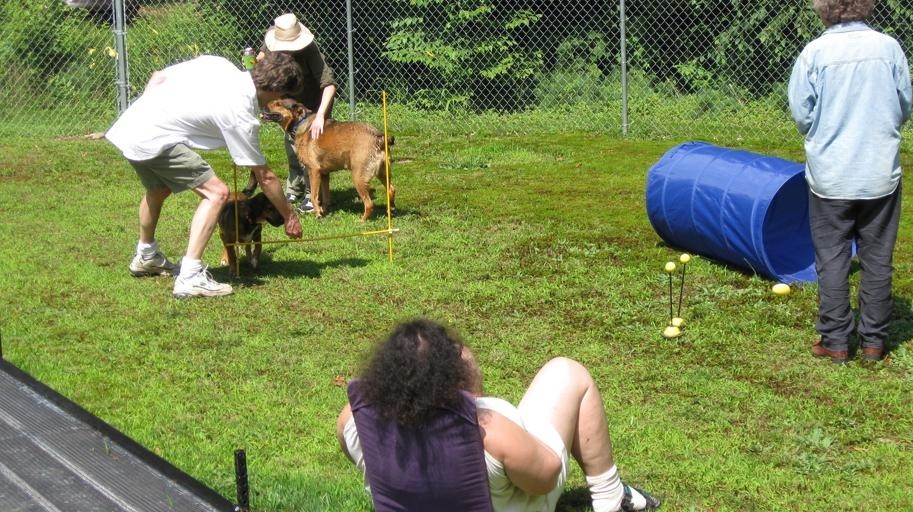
[335,318,662,512]
[787,0,913,364]
[258,13,340,214]
[103,53,302,297]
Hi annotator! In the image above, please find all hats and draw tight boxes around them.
[264,14,315,51]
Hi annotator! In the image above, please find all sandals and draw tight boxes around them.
[591,483,661,512]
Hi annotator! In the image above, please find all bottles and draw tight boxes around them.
[243,49,255,70]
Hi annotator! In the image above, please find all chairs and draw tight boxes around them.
[346,380,493,511]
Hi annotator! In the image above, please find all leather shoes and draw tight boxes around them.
[863,345,883,361]
[812,340,848,363]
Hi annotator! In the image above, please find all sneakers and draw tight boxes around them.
[129,251,180,277]
[174,270,233,297]
[287,193,315,212]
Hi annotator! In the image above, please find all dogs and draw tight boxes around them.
[259,98,395,223]
[218,169,285,278]
